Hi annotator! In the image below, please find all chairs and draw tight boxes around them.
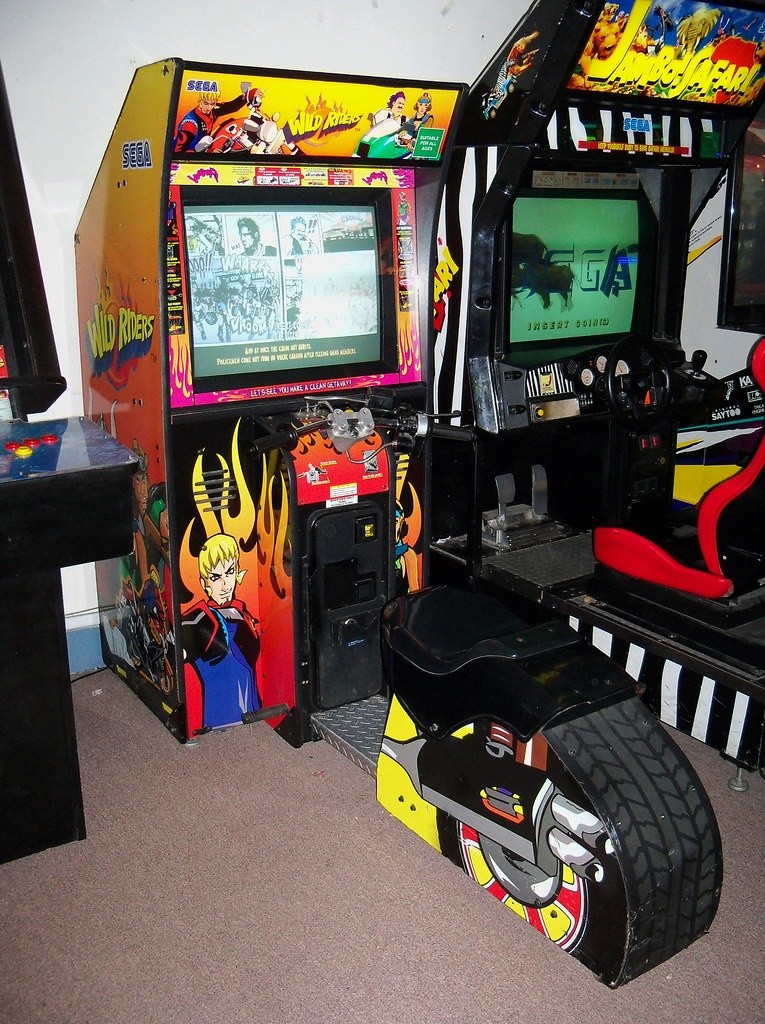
[591,333,765,604]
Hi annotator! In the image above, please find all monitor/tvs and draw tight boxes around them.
[174,185,399,393]
[718,98,765,328]
[505,188,655,354]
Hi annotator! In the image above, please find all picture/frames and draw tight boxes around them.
[717,101,765,328]
